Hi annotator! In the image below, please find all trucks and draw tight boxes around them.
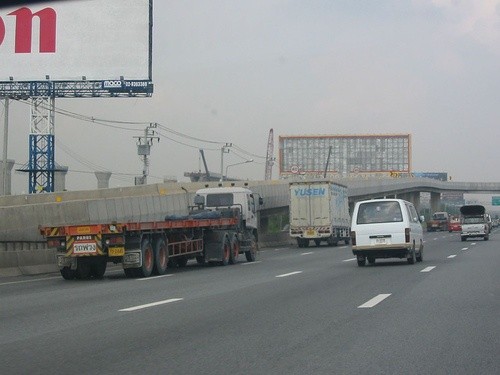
[287,179,351,248]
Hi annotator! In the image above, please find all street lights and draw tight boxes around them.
[226,158,255,180]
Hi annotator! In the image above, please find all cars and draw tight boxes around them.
[426,212,500,233]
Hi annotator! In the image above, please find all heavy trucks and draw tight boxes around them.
[38,186,264,280]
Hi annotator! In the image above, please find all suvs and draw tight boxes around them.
[458,204,490,242]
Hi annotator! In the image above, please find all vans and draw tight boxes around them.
[350,199,425,267]
